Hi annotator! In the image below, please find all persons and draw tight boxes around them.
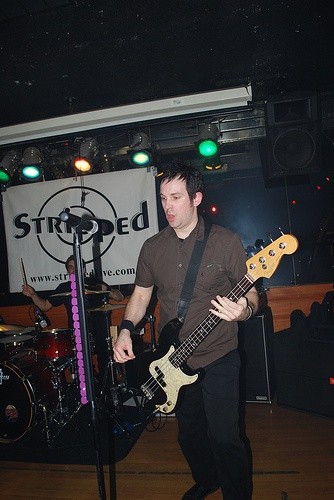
[113,161,259,500]
[22,255,125,375]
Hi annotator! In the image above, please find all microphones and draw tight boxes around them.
[33,306,48,328]
[58,211,99,236]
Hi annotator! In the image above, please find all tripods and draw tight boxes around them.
[90,296,142,410]
[40,366,82,440]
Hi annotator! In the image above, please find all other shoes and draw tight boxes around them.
[181,482,220,500]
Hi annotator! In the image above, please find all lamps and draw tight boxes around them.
[73,135,98,174]
[0,147,22,185]
[21,146,44,181]
[196,115,221,159]
[127,123,153,168]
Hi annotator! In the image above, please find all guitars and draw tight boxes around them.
[125,225,300,415]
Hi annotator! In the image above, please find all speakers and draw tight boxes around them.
[237,314,277,404]
[259,92,334,188]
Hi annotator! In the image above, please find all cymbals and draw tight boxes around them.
[85,303,127,311]
[48,288,111,297]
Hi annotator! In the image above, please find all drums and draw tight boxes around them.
[0,334,36,359]
[0,348,67,442]
[0,323,37,337]
[36,329,75,362]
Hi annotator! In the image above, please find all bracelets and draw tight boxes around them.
[120,320,135,333]
[245,306,253,322]
